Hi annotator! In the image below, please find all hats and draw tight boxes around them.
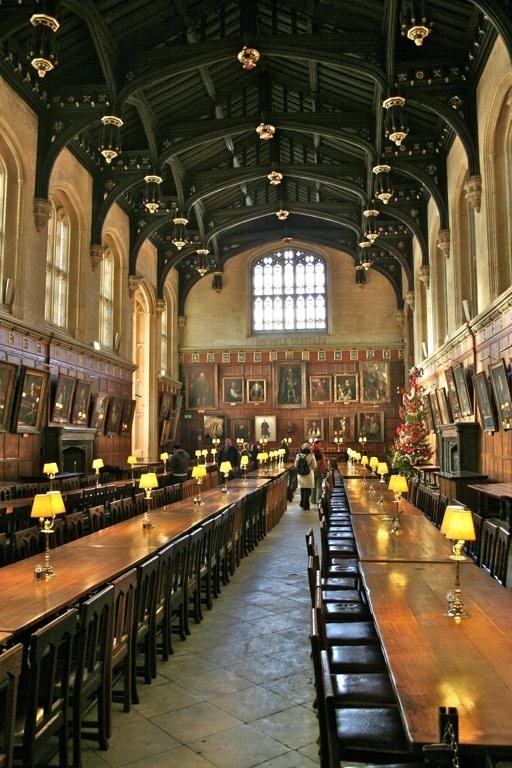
[172,442,181,448]
[314,444,320,451]
[301,443,310,450]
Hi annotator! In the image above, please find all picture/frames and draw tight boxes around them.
[0,361,136,437]
[424,358,512,432]
[185,358,390,444]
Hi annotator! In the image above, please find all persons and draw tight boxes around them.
[295,442,317,511]
[286,367,297,403]
[241,441,263,470]
[190,372,210,406]
[308,421,321,438]
[277,440,290,462]
[229,382,241,401]
[218,438,238,484]
[311,442,326,504]
[339,379,353,400]
[170,442,190,485]
[261,420,269,439]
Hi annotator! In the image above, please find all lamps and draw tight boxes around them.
[399,0,433,46]
[355,96,409,290]
[30,14,60,78]
[237,46,260,70]
[256,123,292,245]
[101,115,223,295]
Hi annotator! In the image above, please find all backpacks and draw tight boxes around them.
[297,454,310,475]
